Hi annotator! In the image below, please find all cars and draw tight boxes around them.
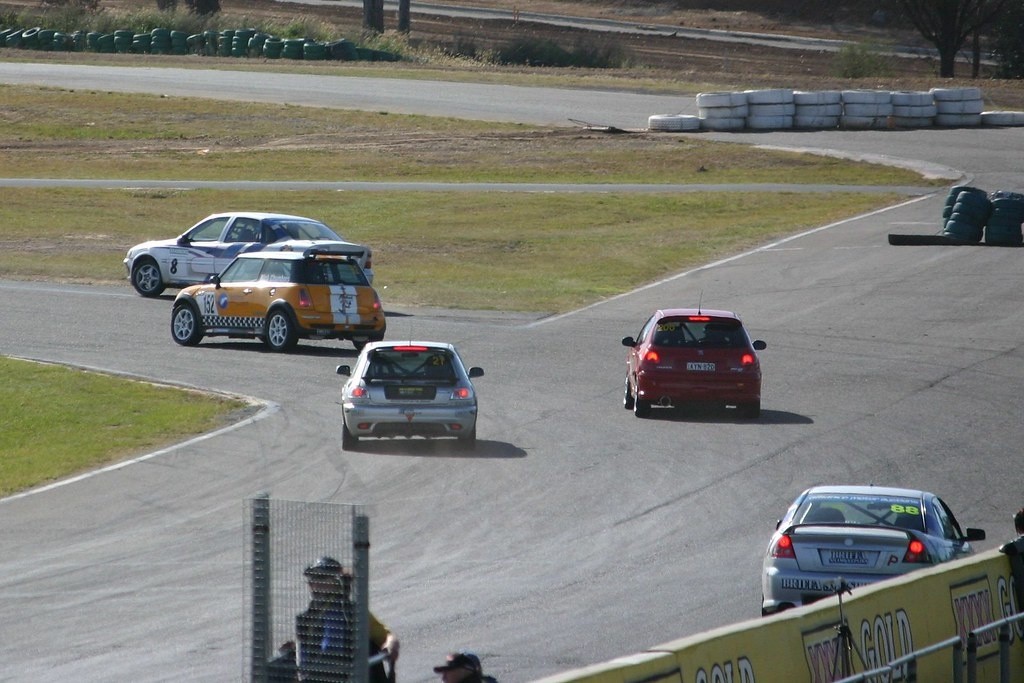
[621,308,767,417]
[336,340,484,452]
[760,485,988,618]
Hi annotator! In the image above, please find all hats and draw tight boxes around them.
[433,652,481,672]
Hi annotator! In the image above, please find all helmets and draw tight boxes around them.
[303,556,341,576]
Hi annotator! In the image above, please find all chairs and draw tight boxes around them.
[265,264,285,277]
[273,228,287,242]
[894,513,924,532]
[703,325,734,344]
[238,229,255,241]
[423,355,443,377]
[810,507,845,523]
[656,329,685,344]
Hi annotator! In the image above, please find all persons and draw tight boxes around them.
[999,508,1024,613]
[296,557,399,683]
[433,653,497,683]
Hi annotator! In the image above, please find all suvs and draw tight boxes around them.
[124,211,375,294]
[170,251,387,352]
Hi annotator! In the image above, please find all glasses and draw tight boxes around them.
[446,653,475,671]
